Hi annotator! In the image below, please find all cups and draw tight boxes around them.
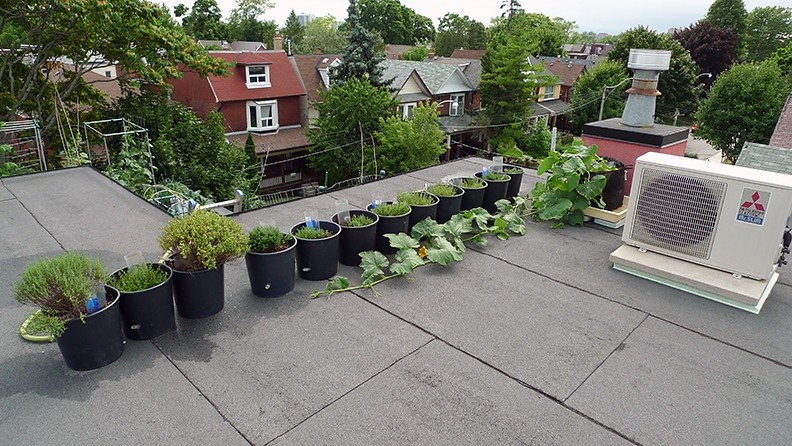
[84,282,106,313]
[453,174,462,186]
[335,199,350,224]
[371,192,387,209]
[304,209,320,231]
[482,165,493,177]
[493,157,503,172]
[260,219,276,227]
[124,250,147,268]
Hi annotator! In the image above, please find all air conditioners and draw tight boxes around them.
[622,151,792,281]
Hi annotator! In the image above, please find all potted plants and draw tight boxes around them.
[366,201,412,252]
[156,210,250,320]
[448,176,488,218]
[489,164,525,204]
[310,137,625,303]
[331,210,379,268]
[423,184,465,225]
[106,263,175,342]
[290,221,341,281]
[396,190,439,234]
[474,170,511,212]
[246,226,297,298]
[9,252,123,372]
